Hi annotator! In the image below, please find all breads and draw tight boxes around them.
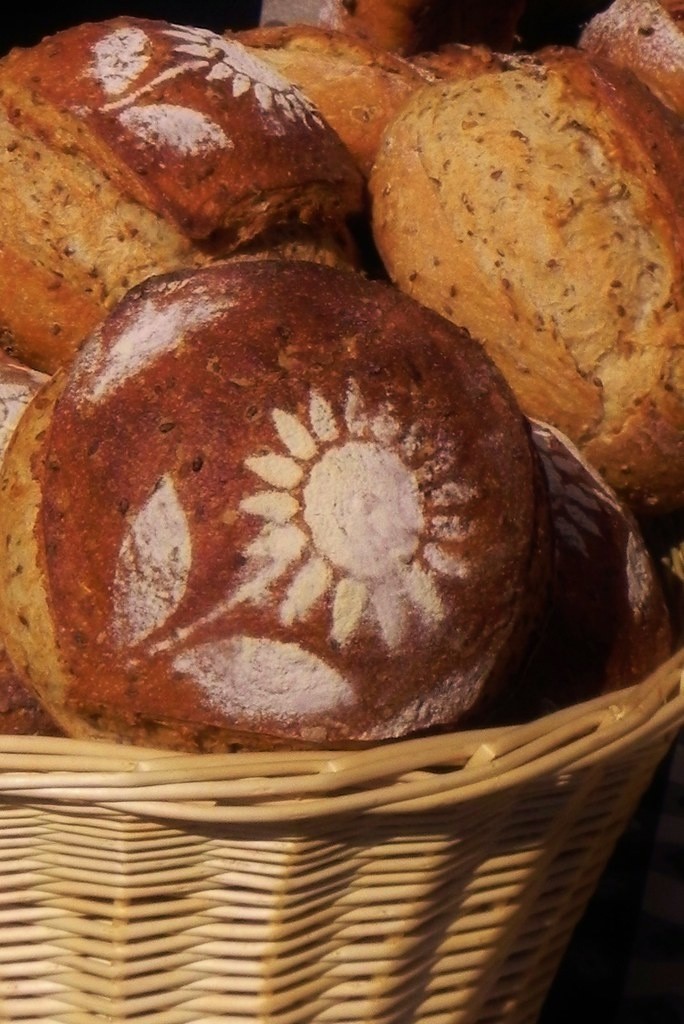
[0,0,684,761]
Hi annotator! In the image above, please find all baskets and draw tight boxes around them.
[0,541,684,1024]
